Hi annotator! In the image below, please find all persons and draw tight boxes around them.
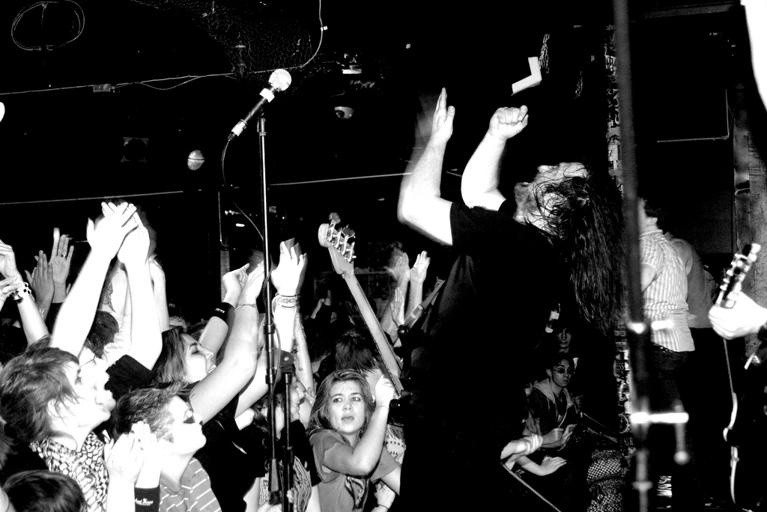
[2,87,766,512]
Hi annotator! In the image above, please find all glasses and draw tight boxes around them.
[553,367,572,373]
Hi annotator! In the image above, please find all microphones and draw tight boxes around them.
[230,67,292,139]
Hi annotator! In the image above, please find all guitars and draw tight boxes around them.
[318,213,425,427]
[714,241,767,506]
